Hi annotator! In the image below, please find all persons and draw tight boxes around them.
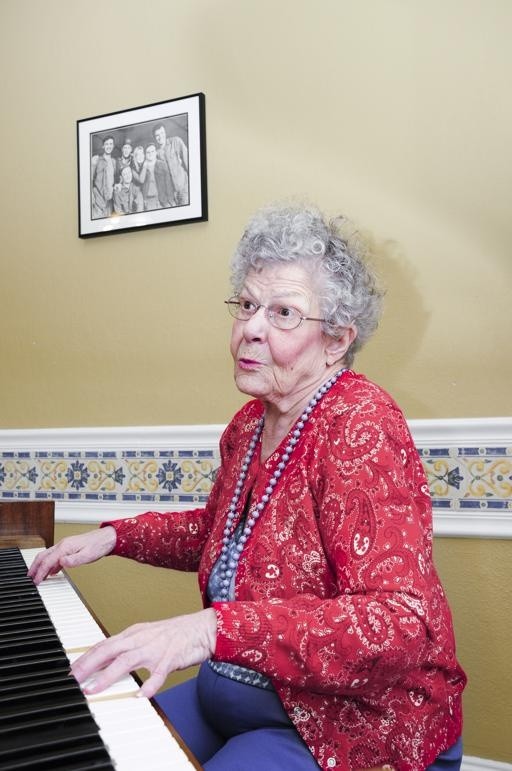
[26,205,470,771]
[89,122,188,219]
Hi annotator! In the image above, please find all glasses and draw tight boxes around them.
[223,296,332,330]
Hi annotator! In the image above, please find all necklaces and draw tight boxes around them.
[220,364,353,602]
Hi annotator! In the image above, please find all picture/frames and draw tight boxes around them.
[76,92,208,239]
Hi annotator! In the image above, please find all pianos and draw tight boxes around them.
[0,502,203,771]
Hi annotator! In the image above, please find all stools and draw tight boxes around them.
[454,751,512,771]
